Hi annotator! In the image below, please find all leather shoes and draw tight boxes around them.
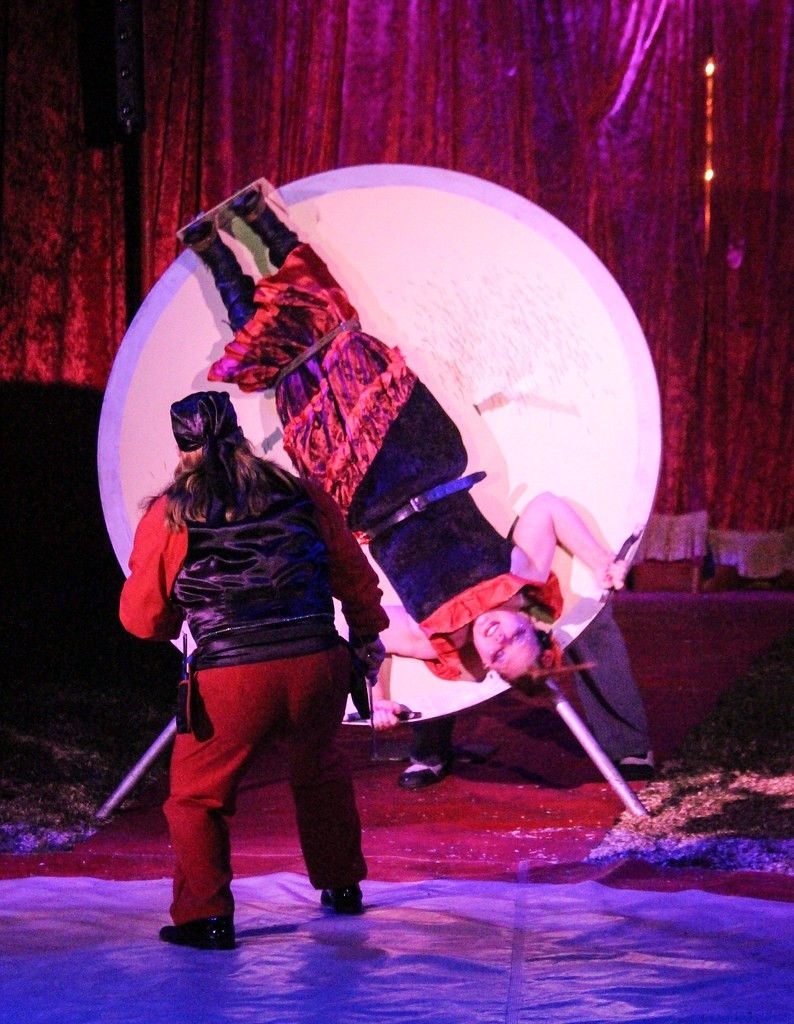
[320,880,364,915]
[160,916,237,951]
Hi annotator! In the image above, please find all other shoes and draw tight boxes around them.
[617,750,657,782]
[398,761,453,789]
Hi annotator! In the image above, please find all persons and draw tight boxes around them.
[398,602,656,791]
[118,392,389,946]
[177,181,632,729]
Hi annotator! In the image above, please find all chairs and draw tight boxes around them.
[631,511,710,596]
[706,528,794,593]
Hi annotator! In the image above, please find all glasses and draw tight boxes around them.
[483,624,538,671]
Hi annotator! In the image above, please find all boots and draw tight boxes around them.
[180,222,244,289]
[228,189,290,248]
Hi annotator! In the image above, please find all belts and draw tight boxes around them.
[366,471,487,541]
[272,320,362,390]
